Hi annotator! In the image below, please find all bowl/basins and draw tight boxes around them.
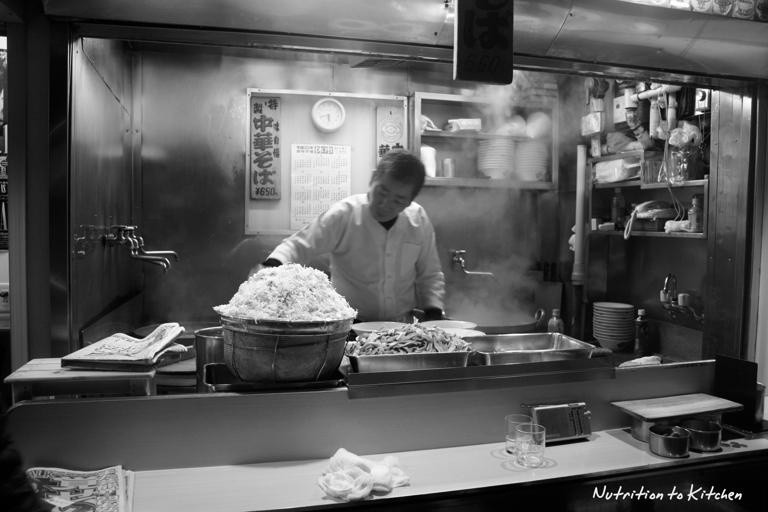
[648,425,690,458]
[420,320,477,330]
[686,421,723,453]
[351,320,408,337]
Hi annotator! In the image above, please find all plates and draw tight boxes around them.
[476,139,549,181]
[591,300,635,351]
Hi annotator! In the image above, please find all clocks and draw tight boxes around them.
[311,98,347,133]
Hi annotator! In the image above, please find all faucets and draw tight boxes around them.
[108,224,181,273]
[453,250,493,279]
[663,273,677,310]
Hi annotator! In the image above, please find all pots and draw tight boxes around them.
[193,326,225,394]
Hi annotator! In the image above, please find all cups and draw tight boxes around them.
[516,423,546,468]
[504,413,532,455]
[441,157,455,177]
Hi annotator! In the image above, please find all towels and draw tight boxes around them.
[318,448,409,501]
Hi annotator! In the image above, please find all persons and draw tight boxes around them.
[248,148,446,341]
[0,408,56,512]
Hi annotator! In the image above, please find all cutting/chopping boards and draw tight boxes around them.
[609,392,745,420]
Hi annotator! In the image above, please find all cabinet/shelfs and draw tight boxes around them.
[586,147,709,240]
[408,91,559,191]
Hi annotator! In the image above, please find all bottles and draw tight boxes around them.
[633,308,647,358]
[547,308,565,333]
[687,198,703,234]
